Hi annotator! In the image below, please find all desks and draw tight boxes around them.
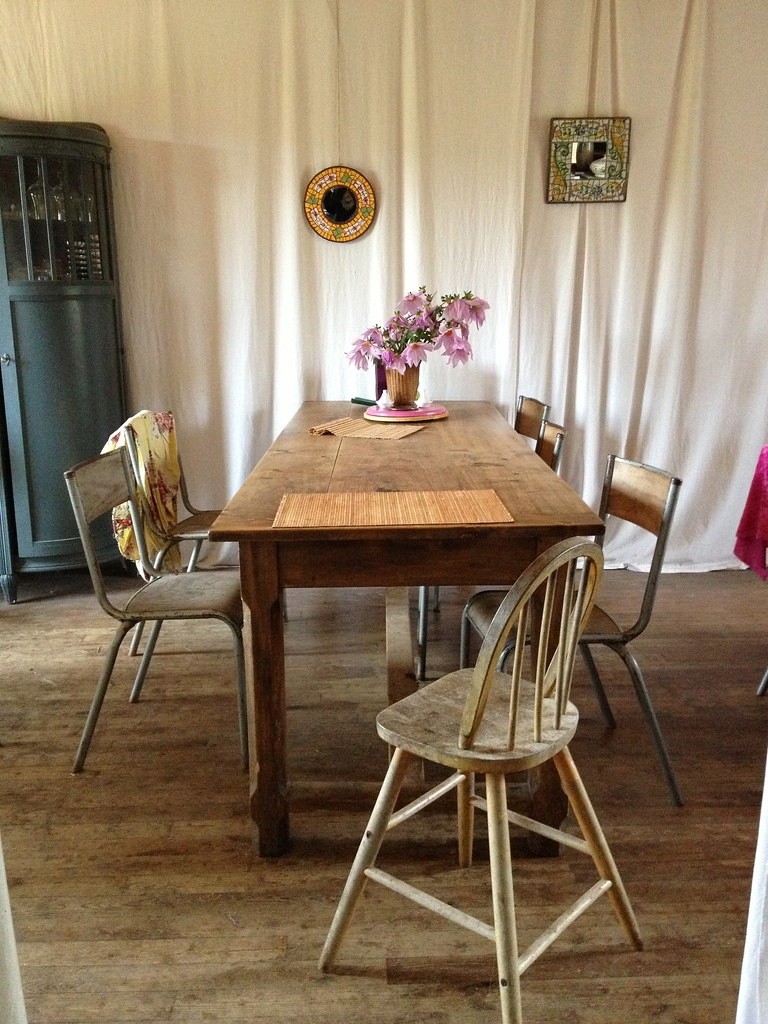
[208,400,606,857]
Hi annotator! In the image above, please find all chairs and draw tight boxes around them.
[461,454,686,810]
[317,534,645,1024]
[416,394,569,681]
[124,411,289,657]
[62,444,251,775]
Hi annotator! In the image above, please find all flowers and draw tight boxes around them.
[344,284,491,375]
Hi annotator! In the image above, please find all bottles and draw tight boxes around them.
[26,160,93,222]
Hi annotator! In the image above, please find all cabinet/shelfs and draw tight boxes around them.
[0,116,139,603]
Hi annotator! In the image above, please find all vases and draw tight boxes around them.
[385,359,421,411]
